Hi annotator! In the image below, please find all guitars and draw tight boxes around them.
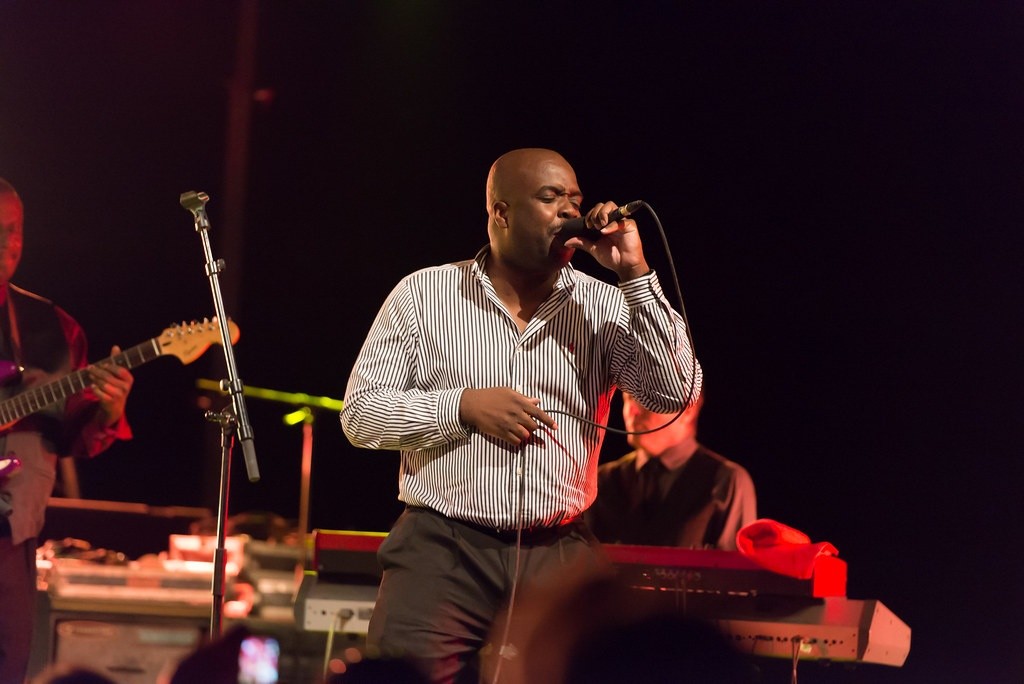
[0,314,241,491]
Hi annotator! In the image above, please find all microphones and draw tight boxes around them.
[562,199,644,244]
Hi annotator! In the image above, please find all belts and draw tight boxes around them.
[460,518,572,541]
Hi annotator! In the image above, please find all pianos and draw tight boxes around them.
[288,520,915,669]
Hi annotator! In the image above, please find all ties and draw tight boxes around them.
[644,459,668,500]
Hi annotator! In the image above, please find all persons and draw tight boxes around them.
[593,376,761,555]
[41,609,758,684]
[341,149,702,684]
[0,171,134,684]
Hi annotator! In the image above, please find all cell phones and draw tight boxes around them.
[237,631,280,684]
